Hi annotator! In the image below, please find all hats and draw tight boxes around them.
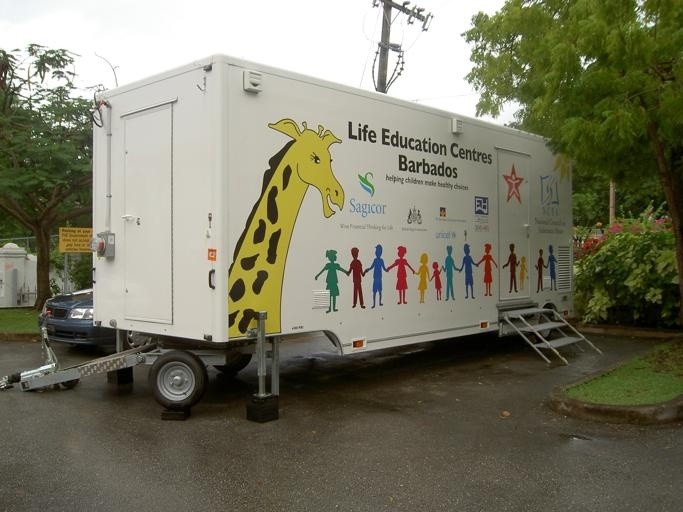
[38,290,154,355]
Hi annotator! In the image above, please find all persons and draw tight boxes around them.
[535,248,547,293]
[442,245,459,301]
[430,262,444,301]
[386,246,415,305]
[314,249,348,312]
[519,258,527,290]
[413,253,430,302]
[546,246,559,291]
[476,243,498,297]
[366,245,388,308]
[346,247,366,309]
[503,243,519,294]
[459,245,479,298]
